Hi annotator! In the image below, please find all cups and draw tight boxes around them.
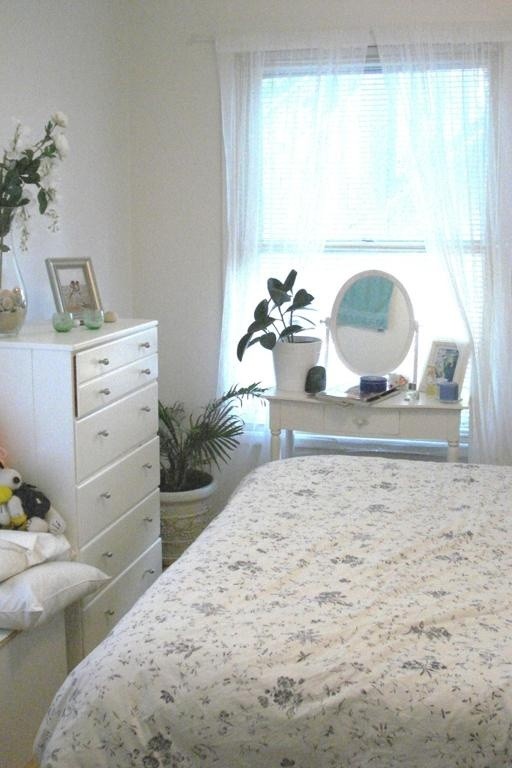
[438,382,459,403]
[52,310,73,333]
[83,309,105,331]
[360,375,388,401]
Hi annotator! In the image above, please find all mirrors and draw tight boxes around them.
[330,270,414,376]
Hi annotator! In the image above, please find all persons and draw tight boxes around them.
[66,280,85,308]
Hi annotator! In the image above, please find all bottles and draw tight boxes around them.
[405,382,422,405]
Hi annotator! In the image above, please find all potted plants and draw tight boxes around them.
[236,269,321,391]
[158,381,268,566]
[0,112,69,336]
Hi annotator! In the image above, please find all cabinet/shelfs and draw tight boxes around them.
[1,313,162,673]
[265,390,469,463]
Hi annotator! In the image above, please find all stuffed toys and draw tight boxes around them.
[0,449,68,535]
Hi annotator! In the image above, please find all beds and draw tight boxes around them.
[32,454,511,768]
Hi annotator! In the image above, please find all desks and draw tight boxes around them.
[0,609,69,766]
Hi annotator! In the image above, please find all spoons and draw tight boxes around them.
[366,384,404,402]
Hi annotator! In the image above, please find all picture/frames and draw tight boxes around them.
[46,258,104,325]
[418,341,471,397]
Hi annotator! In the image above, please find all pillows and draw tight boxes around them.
[1,530,112,631]
